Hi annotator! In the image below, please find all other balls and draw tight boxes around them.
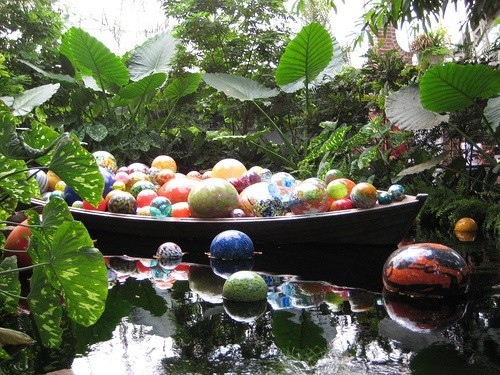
[222,270,266,301]
[210,229,254,261]
[455,218,478,231]
[156,242,180,260]
[26,148,406,218]
[430,167,454,192]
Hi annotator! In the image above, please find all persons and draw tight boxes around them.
[352,102,408,159]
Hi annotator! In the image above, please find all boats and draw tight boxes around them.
[14,165,427,266]
